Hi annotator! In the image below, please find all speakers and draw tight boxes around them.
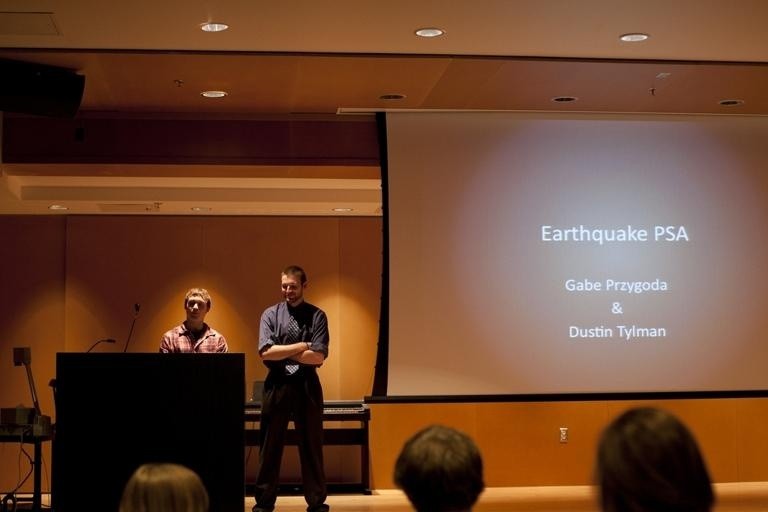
[0,57,87,121]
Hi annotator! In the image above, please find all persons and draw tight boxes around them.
[392,422,485,512]
[120,463,210,511]
[160,286,229,353]
[253,264,329,512]
[591,405,718,512]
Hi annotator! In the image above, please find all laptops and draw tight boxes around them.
[244,379,266,408]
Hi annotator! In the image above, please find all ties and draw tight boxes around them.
[283,310,300,375]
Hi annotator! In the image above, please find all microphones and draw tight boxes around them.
[87,337,116,353]
[123,302,141,353]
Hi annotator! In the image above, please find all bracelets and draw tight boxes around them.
[305,342,310,350]
